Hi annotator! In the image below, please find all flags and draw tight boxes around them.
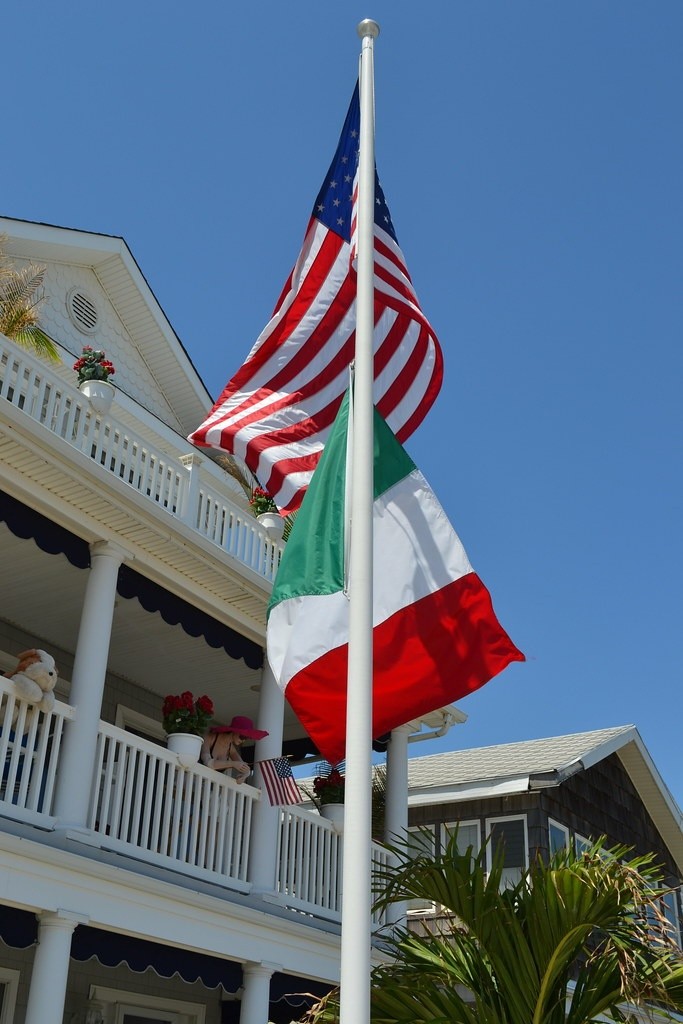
[181,76,444,540]
[264,374,530,767]
[259,757,303,808]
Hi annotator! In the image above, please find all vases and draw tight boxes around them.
[319,804,344,834]
[165,733,205,769]
[78,379,116,413]
[256,511,286,541]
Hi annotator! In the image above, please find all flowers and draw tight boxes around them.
[312,768,346,804]
[73,343,115,387]
[249,485,279,516]
[162,691,216,739]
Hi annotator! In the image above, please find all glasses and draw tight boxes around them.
[239,735,245,740]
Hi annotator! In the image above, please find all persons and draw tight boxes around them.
[200,714,257,786]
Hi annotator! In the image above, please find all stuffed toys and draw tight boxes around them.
[0,648,60,735]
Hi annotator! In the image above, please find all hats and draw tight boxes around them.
[211,716,268,740]
